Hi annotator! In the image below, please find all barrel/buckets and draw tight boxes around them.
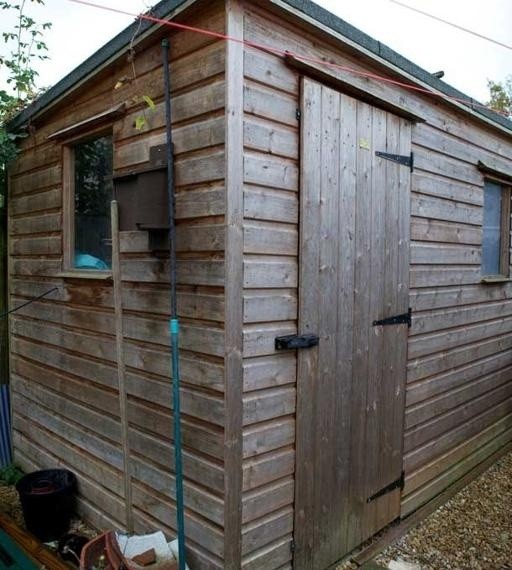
[14,468,79,544]
